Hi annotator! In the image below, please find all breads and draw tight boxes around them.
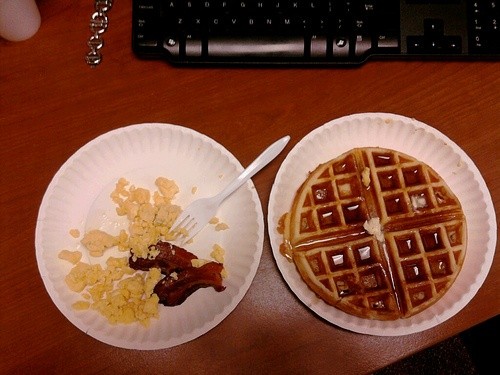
[287,147,467,320]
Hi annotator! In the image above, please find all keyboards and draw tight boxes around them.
[131,0,500,71]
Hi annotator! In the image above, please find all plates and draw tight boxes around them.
[267,112,498,337]
[35,122,264,350]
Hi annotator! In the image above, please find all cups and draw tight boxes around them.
[0,0,41,42]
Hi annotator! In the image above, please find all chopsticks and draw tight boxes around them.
[167,135,291,245]
